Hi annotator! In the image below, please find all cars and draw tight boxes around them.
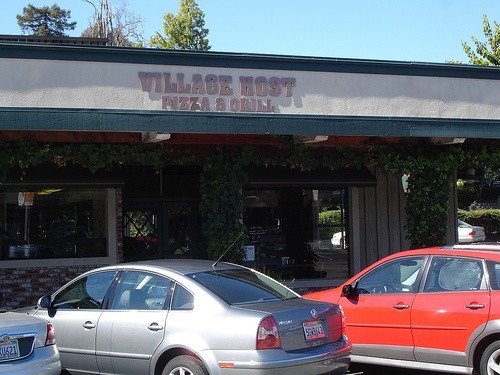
[457,218,486,243]
[302,243,500,375]
[0,309,64,375]
[8,258,352,375]
